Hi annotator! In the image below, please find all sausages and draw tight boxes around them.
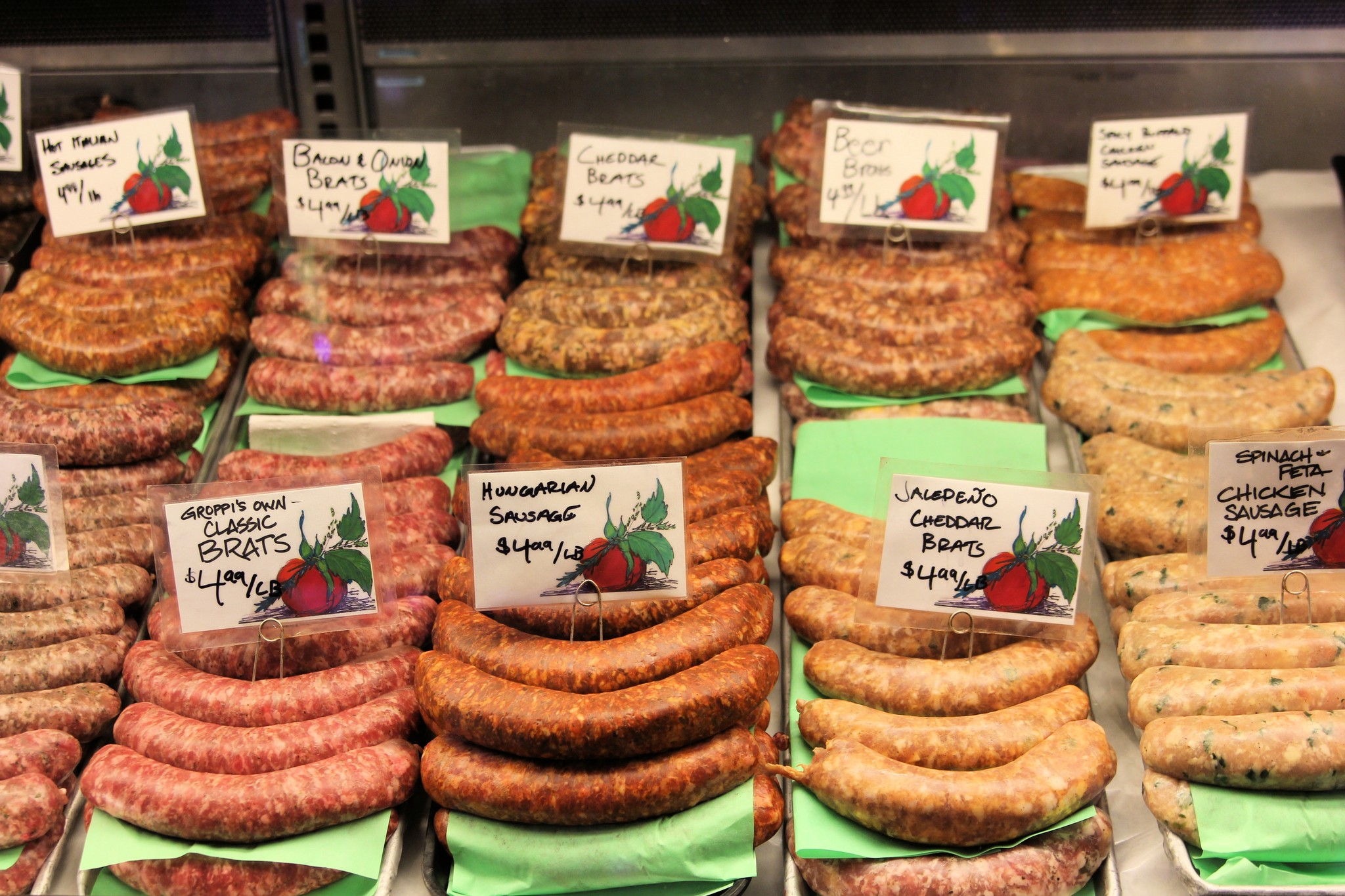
[0,108,1345,896]
